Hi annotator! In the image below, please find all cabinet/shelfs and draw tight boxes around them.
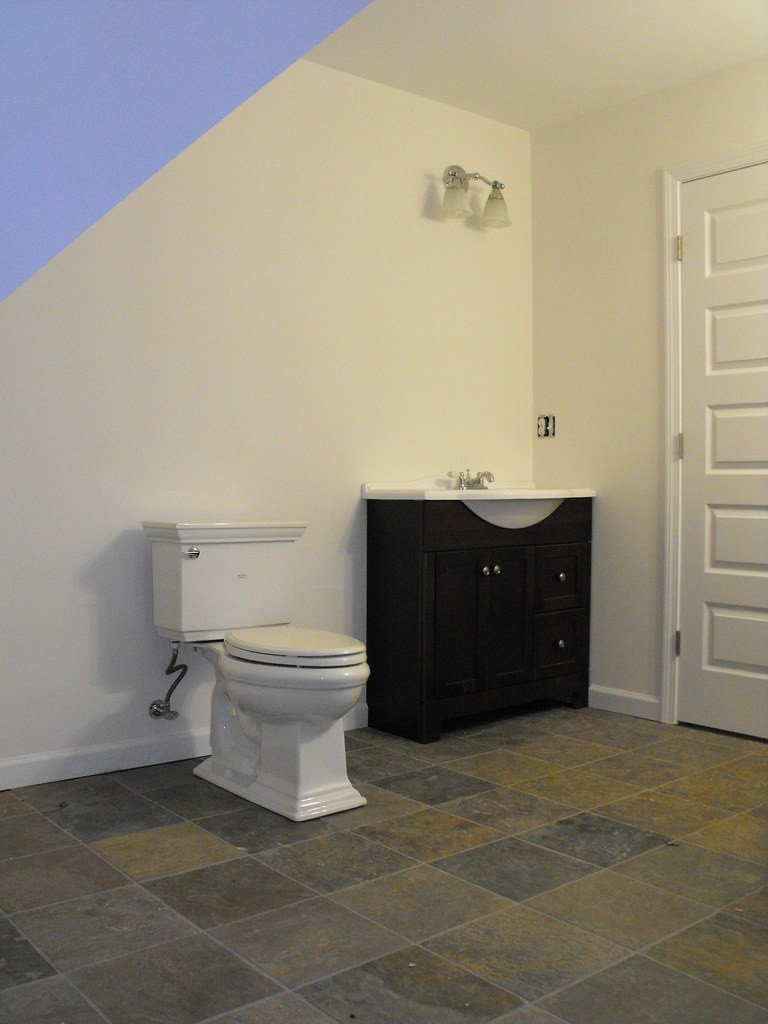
[366,497,593,743]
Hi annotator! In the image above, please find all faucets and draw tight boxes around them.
[466,471,495,490]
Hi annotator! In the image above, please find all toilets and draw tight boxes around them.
[140,518,373,825]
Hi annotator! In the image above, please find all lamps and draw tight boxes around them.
[439,165,512,229]
